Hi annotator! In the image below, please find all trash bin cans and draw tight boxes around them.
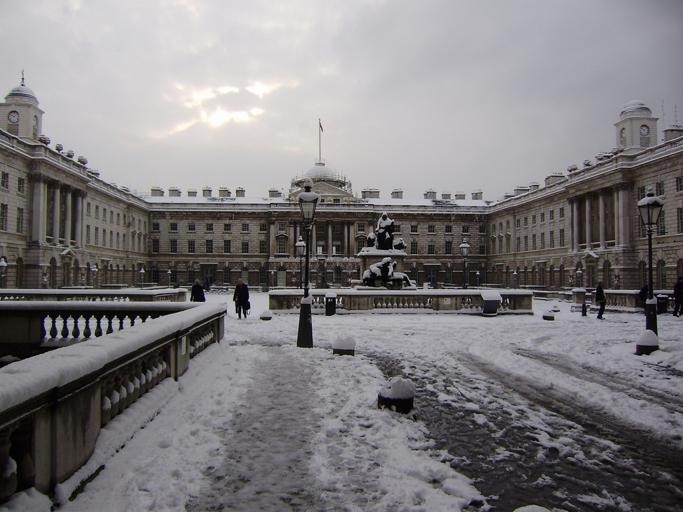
[326,293,335,316]
[657,295,667,314]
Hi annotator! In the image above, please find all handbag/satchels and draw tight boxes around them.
[245,302,250,309]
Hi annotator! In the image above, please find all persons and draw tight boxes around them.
[638,284,648,309]
[594,279,605,321]
[189,277,205,303]
[374,211,395,241]
[672,276,683,317]
[232,277,248,321]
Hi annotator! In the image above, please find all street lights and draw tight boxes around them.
[635,190,664,355]
[459,237,470,289]
[297,178,320,348]
[295,235,306,289]
